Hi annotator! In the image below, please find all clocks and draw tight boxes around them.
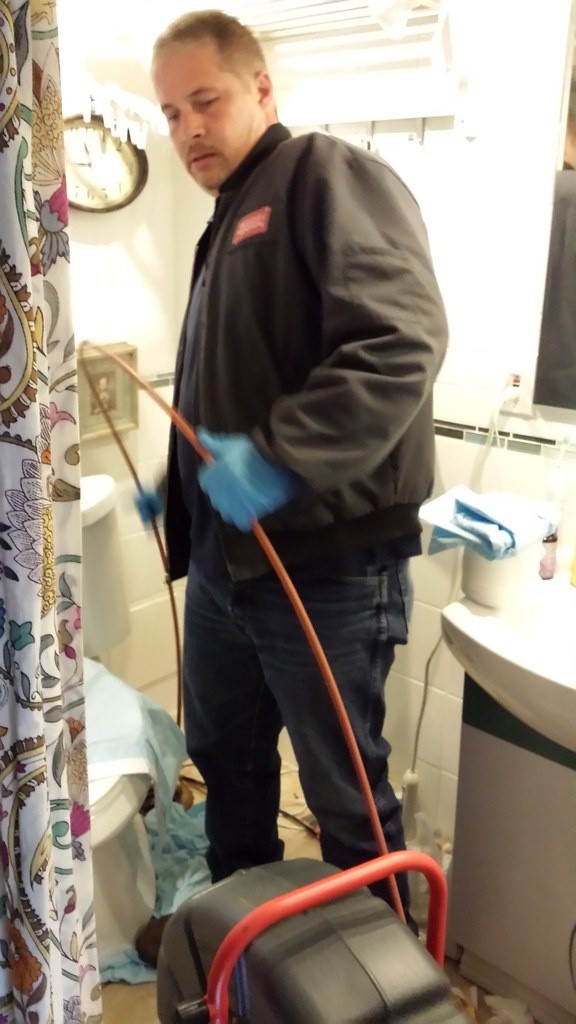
[63,115,148,212]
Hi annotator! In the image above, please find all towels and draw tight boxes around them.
[98,797,210,986]
[418,483,558,562]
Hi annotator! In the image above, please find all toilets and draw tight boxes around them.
[82,470,157,955]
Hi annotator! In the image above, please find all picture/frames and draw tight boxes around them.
[76,342,139,441]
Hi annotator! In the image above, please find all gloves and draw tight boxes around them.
[191,428,304,534]
[134,492,163,524]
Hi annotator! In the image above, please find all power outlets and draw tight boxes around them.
[500,368,535,417]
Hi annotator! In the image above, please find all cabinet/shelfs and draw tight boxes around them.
[443,669,576,1024]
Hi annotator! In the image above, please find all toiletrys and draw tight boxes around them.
[539,523,576,588]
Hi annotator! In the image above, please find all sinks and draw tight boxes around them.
[440,561,576,753]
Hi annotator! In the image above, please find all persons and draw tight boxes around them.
[533,72,576,409]
[136,9,448,974]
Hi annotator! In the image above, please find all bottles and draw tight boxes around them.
[539,527,558,580]
